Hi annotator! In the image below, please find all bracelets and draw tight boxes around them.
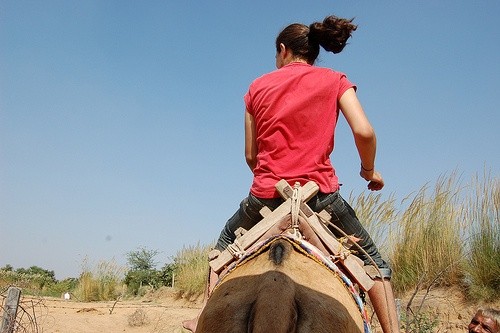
[361,163,374,171]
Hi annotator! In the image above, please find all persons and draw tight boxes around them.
[183,15,400,333]
